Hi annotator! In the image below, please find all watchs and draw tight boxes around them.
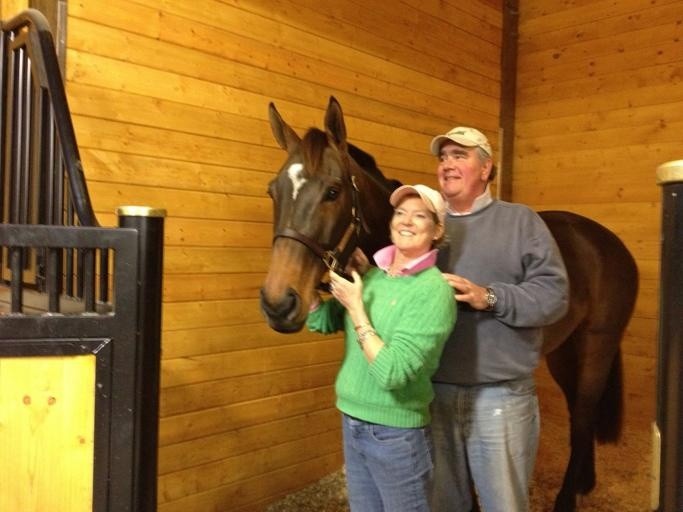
[486,287,497,312]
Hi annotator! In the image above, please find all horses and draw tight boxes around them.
[259,95,640,512]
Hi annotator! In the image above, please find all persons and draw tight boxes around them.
[343,126,569,511]
[307,185,457,512]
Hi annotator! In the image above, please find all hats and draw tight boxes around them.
[390,184,447,227]
[431,126,492,155]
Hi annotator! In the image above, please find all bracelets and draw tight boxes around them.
[353,321,376,350]
